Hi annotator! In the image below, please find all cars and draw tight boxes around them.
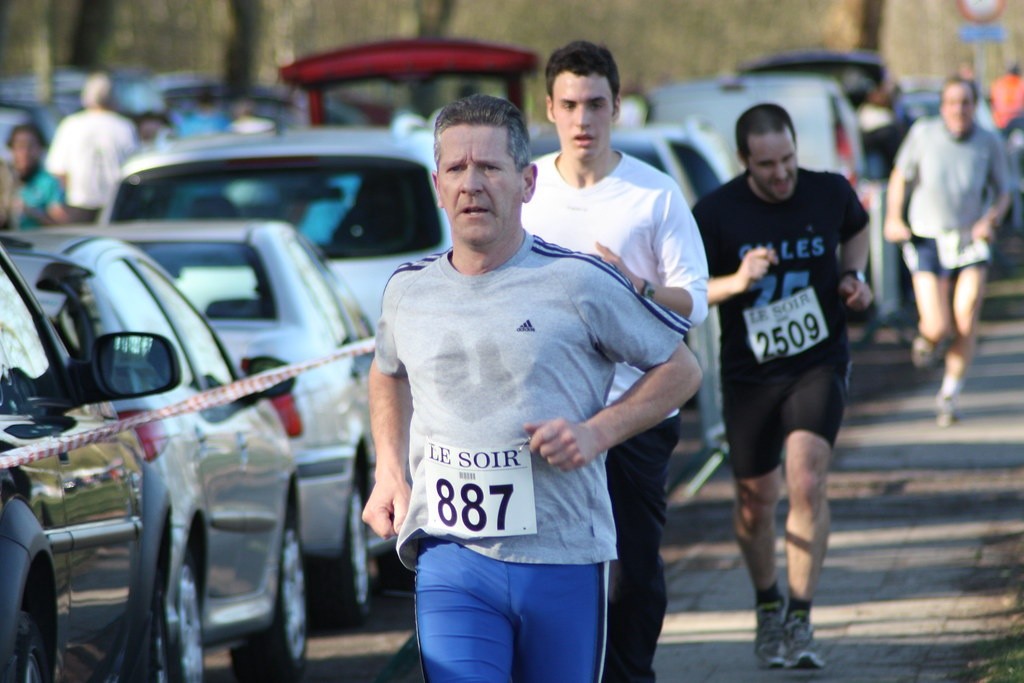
[0,237,186,683]
[1,227,311,683]
[0,33,1024,416]
[21,216,416,630]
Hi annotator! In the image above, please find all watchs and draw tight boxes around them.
[641,279,656,301]
[841,270,866,282]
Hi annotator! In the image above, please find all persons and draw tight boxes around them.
[0,67,272,237]
[362,94,703,683]
[691,102,877,666]
[520,39,710,683]
[864,55,1024,425]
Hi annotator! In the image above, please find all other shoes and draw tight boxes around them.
[911,334,933,369]
[935,392,954,426]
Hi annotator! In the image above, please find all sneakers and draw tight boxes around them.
[782,611,823,668]
[755,599,786,665]
